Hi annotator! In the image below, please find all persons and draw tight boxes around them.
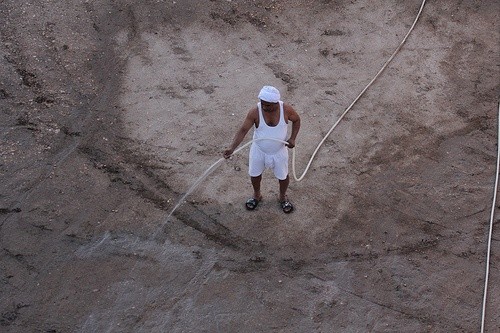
[222,85,303,213]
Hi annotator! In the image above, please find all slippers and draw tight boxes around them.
[281,198,293,213]
[246,195,262,209]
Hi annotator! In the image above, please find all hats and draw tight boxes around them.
[258,85,280,103]
